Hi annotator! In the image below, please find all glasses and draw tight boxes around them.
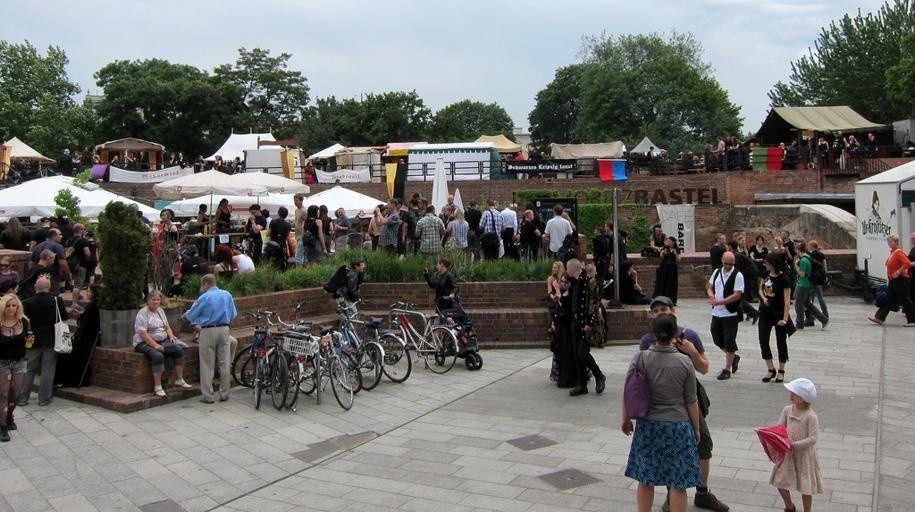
[763,261,770,265]
[722,259,736,266]
[0,264,11,267]
[561,280,569,284]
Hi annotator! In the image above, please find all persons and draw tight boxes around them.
[132,278,238,403]
[306,159,314,168]
[906,232,915,284]
[621,128,915,177]
[341,260,366,338]
[868,190,882,224]
[0,217,106,441]
[768,377,824,512]
[622,313,702,511]
[639,296,729,512]
[706,229,829,383]
[868,235,915,328]
[545,223,681,396]
[424,259,472,352]
[364,194,576,263]
[515,151,524,161]
[3,147,150,186]
[162,150,246,175]
[132,193,363,295]
[315,157,326,171]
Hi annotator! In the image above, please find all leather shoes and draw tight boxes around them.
[568,385,589,395]
[218,395,230,401]
[174,379,193,389]
[776,369,785,383]
[596,373,607,393]
[153,389,167,397]
[199,397,215,404]
[761,368,776,382]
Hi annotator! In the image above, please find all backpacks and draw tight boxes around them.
[810,258,827,286]
[302,230,316,248]
[323,264,347,293]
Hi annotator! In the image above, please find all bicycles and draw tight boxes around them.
[230,300,462,411]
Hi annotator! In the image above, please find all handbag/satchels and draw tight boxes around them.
[174,338,188,348]
[696,378,711,418]
[479,232,501,246]
[738,307,744,322]
[787,314,796,337]
[623,372,652,419]
[875,289,900,313]
[587,320,606,346]
[52,321,73,355]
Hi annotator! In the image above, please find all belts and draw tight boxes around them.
[200,323,229,328]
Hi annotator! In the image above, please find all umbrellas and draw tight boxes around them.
[0,135,56,166]
[753,424,793,465]
[153,166,388,221]
[307,142,349,160]
[431,156,450,217]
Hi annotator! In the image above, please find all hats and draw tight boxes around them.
[186,244,199,254]
[783,377,818,405]
[650,295,674,308]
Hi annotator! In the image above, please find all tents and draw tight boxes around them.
[335,145,390,185]
[380,142,499,182]
[549,141,628,179]
[1,173,164,224]
[202,132,287,177]
[471,134,530,161]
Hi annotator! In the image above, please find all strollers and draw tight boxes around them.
[434,301,486,374]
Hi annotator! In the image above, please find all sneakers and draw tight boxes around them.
[902,322,915,328]
[70,304,85,314]
[795,324,805,329]
[867,316,884,325]
[752,309,759,323]
[0,426,9,441]
[716,369,731,380]
[732,354,741,373]
[804,320,815,327]
[694,492,730,512]
[745,313,752,321]
[783,504,796,512]
[38,397,53,405]
[6,418,16,430]
[16,401,27,406]
[661,498,670,512]
[822,318,830,328]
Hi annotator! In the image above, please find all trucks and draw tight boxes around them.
[853,159,914,303]
